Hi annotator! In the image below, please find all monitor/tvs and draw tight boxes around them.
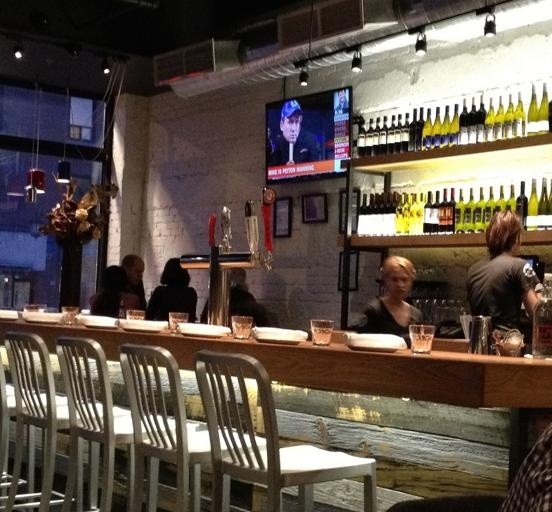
[517,255,538,272]
[266,85,352,185]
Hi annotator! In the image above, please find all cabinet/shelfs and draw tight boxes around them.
[340,101,552,332]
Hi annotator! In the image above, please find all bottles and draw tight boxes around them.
[357,82,552,157]
[532,272,551,360]
[357,176,552,237]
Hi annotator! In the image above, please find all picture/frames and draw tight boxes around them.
[339,187,360,234]
[338,251,360,292]
[301,193,328,224]
[273,196,292,238]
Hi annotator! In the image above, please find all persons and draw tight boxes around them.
[90,265,142,321]
[201,268,250,326]
[358,254,425,334]
[465,210,544,356]
[145,257,197,323]
[268,99,314,166]
[119,253,149,310]
[496,421,552,512]
[335,88,350,116]
[229,291,283,327]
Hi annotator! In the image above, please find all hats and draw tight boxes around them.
[281,100,304,118]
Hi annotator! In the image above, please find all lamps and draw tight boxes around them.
[293,0,518,86]
[0,26,130,75]
[6,44,81,203]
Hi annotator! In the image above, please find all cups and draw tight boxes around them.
[309,320,334,346]
[60,306,80,326]
[167,312,189,333]
[409,323,435,355]
[409,297,467,329]
[231,315,252,339]
[126,309,146,322]
[467,315,494,355]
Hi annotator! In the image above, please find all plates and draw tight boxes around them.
[252,326,407,352]
[0,309,232,345]
